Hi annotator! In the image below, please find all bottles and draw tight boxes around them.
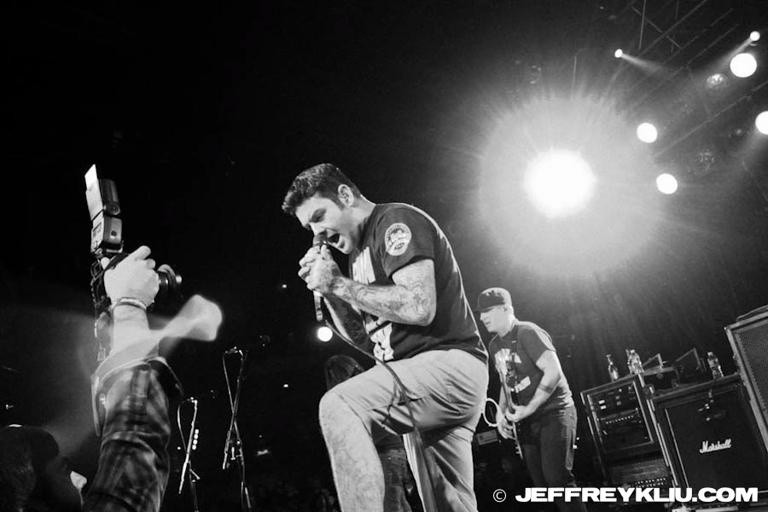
[606,354,618,381]
[628,349,644,374]
[707,352,724,381]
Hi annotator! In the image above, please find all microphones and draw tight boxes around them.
[312,235,328,323]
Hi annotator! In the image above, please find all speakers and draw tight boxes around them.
[651,374,768,512]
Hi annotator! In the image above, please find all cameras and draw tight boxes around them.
[84,163,182,311]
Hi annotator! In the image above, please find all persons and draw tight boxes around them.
[322,349,416,512]
[0,243,173,510]
[474,285,591,512]
[279,164,492,511]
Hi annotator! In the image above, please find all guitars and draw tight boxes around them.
[501,359,524,462]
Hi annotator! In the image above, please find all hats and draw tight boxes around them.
[472,287,511,313]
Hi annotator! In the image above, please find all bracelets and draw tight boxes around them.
[107,296,148,316]
